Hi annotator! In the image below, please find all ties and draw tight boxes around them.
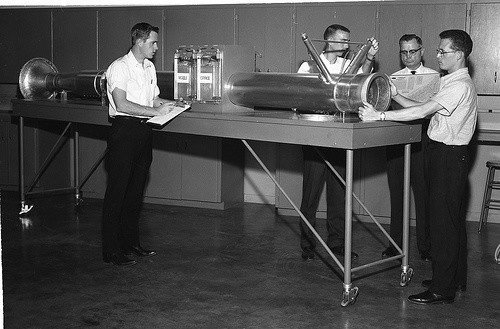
[411,71,416,74]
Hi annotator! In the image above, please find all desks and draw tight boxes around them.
[12,98,430,306]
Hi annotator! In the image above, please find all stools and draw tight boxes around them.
[478,159,500,234]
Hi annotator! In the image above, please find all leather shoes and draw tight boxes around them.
[422,279,466,292]
[127,244,156,257]
[332,250,358,259]
[407,290,456,305]
[302,247,314,261]
[381,246,398,257]
[103,252,136,265]
[426,250,433,261]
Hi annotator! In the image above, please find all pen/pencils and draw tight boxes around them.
[161,100,165,104]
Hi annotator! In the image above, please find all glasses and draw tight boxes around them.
[436,49,457,55]
[400,47,422,56]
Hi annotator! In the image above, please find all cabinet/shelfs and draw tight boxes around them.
[0,0,500,224]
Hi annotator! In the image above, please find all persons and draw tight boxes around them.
[382,33,441,262]
[358,29,478,306]
[296,24,380,261]
[101,22,189,265]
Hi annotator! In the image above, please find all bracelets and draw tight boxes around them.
[367,56,375,62]
[380,112,385,121]
